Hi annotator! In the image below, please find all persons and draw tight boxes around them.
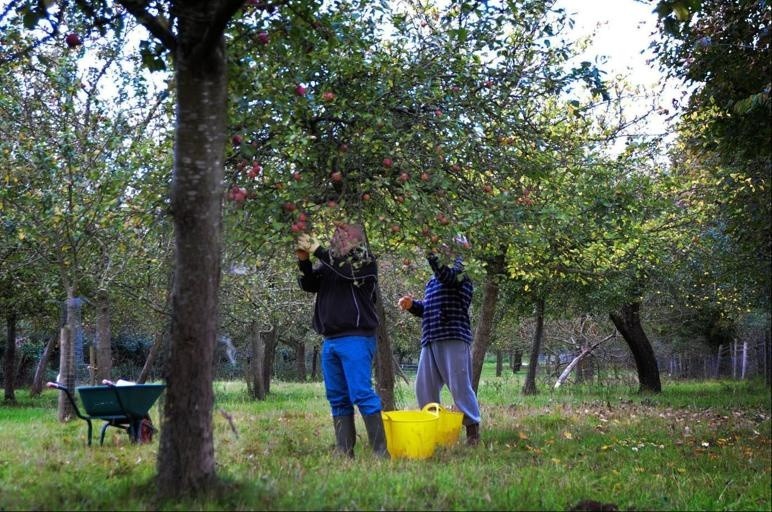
[294,224,391,461]
[398,234,481,448]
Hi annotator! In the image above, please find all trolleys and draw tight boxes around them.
[46,379,167,448]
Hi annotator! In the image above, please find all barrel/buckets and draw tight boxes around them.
[379,402,439,462]
[437,404,464,451]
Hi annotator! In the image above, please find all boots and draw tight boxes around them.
[362,410,392,463]
[463,422,483,449]
[328,413,356,461]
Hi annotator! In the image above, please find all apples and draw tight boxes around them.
[67,35,83,48]
[226,33,532,265]
